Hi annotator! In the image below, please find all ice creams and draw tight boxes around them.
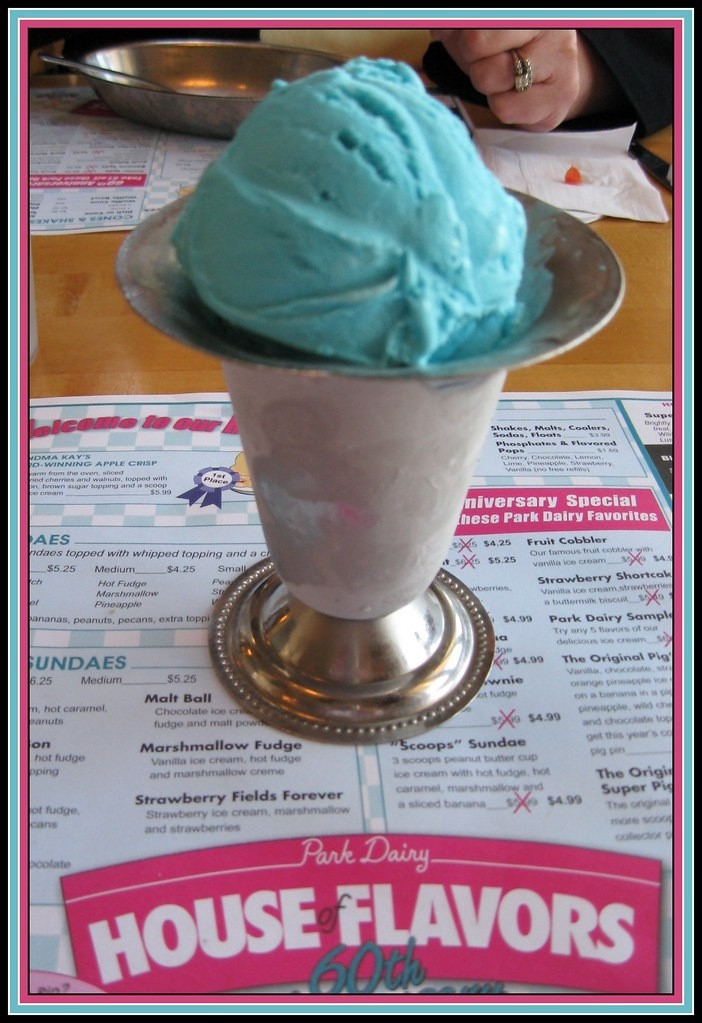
[172,53,530,370]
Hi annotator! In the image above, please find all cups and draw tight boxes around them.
[111,178,628,743]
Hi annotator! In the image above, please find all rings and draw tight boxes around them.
[510,48,532,92]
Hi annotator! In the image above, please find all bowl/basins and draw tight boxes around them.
[76,38,349,138]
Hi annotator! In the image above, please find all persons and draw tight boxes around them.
[420,30,672,141]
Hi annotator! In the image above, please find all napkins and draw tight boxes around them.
[467,120,668,229]
